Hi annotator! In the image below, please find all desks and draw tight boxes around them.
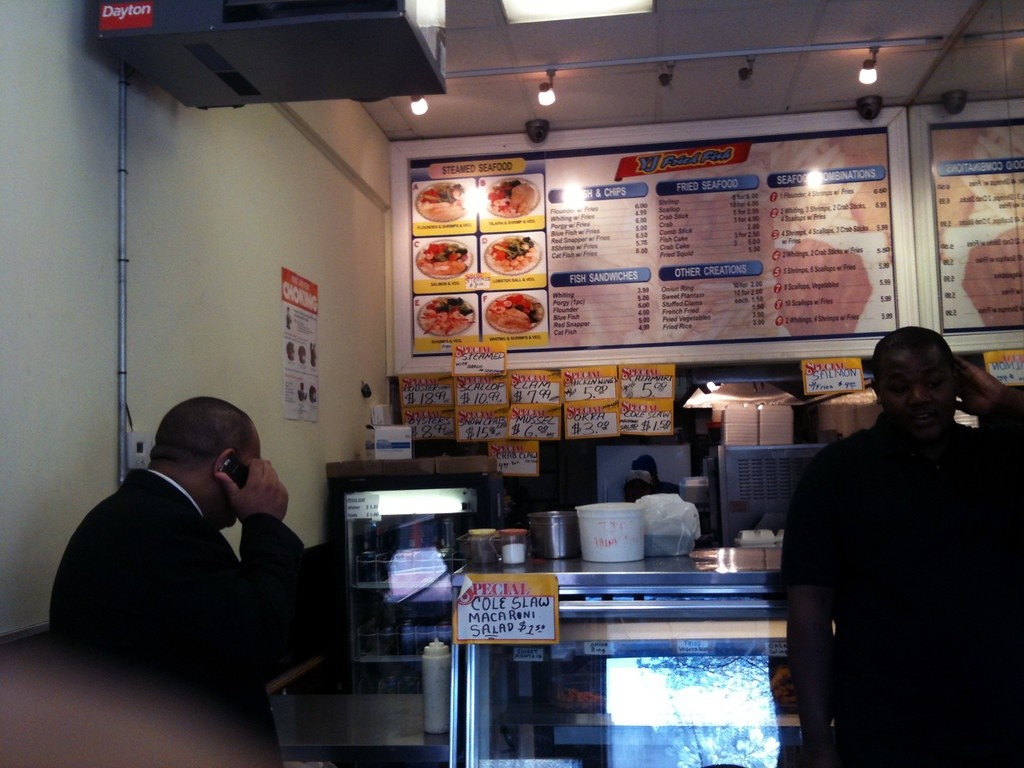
[267,692,449,764]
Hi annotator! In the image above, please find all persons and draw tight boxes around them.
[621,453,679,503]
[51,398,350,768]
[780,325,1024,768]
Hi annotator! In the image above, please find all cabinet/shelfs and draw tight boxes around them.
[445,547,802,768]
[325,456,504,697]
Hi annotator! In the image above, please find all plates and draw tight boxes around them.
[416,182,465,221]
[415,240,473,279]
[487,177,539,218]
[418,297,476,336]
[484,236,542,275]
[486,293,545,333]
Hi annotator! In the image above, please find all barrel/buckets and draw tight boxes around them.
[575,502,645,562]
[528,511,578,558]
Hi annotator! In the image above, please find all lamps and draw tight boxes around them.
[737,55,755,82]
[659,61,675,87]
[410,94,430,118]
[537,70,558,107]
[858,46,879,85]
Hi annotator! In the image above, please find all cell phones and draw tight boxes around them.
[219,458,250,489]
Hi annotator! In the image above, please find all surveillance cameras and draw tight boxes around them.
[942,89,968,114]
[525,119,549,143]
[857,94,883,120]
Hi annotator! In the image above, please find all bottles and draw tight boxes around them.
[422,638,451,734]
